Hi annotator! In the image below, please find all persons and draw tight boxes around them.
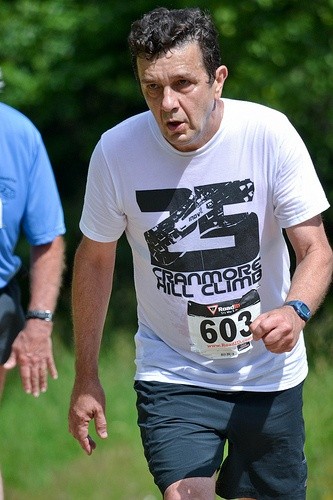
[0,74,66,399]
[69,8,333,500]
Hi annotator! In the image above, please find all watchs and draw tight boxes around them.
[283,301,312,324]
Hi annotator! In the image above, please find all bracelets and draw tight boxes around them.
[25,310,54,321]
